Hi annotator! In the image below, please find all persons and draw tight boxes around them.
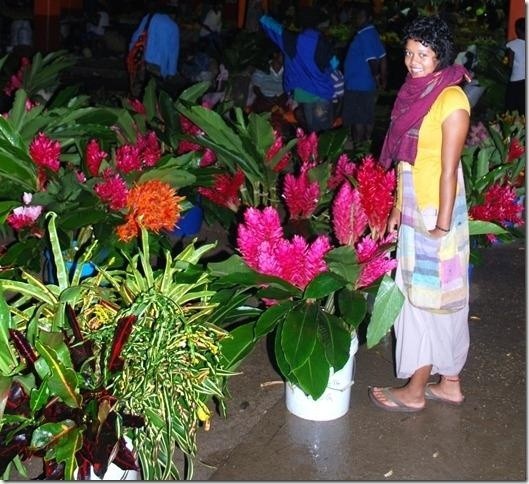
[367,11,473,413]
[0,0,526,160]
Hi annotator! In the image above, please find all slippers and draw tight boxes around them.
[369,387,423,412]
[424,382,466,406]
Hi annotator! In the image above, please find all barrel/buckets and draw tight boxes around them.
[285,330,360,424]
[46,239,95,281]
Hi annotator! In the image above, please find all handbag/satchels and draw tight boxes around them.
[394,204,470,314]
[126,33,147,73]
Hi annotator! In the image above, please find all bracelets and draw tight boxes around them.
[434,223,451,232]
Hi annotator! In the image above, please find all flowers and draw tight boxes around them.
[174,92,408,412]
[458,108,527,251]
[0,51,254,483]
